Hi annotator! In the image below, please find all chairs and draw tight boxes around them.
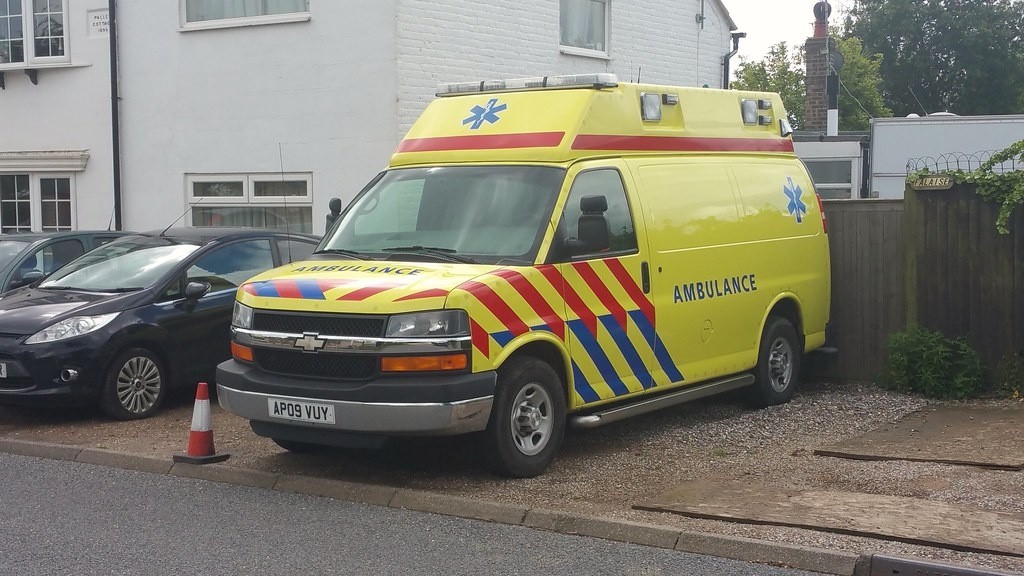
[578,195,611,255]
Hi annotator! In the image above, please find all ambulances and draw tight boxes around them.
[215,72,831,480]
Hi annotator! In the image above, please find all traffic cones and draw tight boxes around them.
[173,382,231,465]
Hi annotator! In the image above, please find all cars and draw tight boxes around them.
[0,227,323,421]
[0,230,138,297]
